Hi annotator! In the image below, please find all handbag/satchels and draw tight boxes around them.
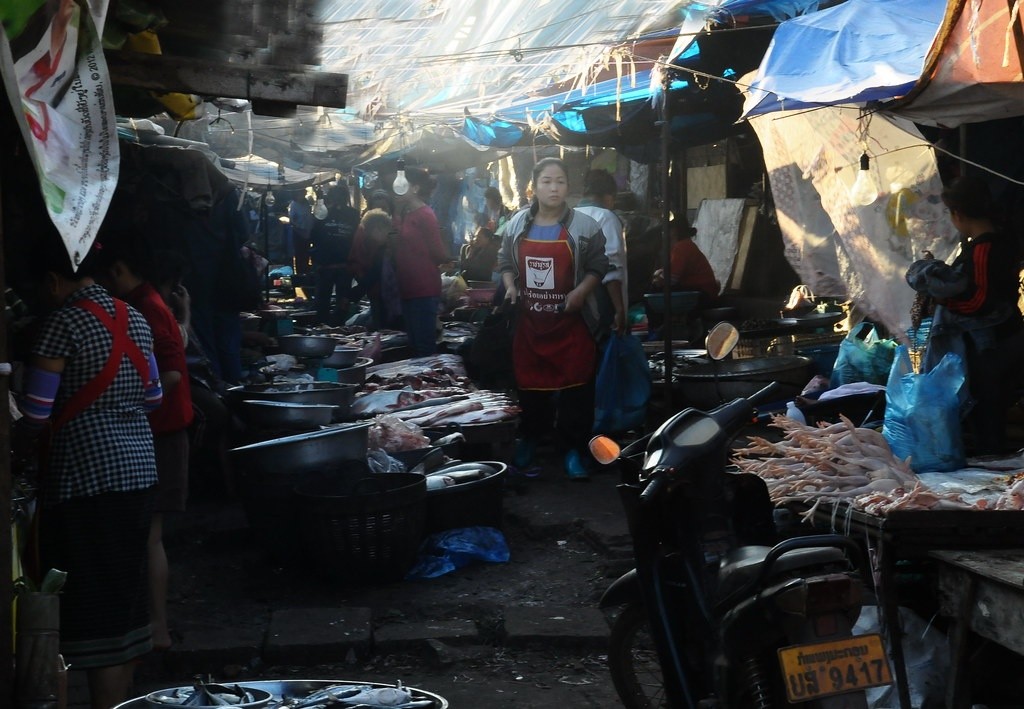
[832,320,967,473]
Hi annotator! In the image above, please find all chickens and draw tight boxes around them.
[728,410,1024,527]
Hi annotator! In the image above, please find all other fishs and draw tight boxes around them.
[236,325,523,492]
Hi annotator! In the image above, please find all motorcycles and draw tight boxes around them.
[616,380,903,709]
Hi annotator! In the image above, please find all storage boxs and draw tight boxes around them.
[209,250,1024,709]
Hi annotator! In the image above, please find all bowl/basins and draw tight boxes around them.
[230,280,811,518]
[114,674,451,709]
[146,686,274,708]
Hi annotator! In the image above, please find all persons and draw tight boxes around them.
[649,215,721,311]
[87,228,194,658]
[397,168,452,358]
[20,223,165,708]
[498,157,610,480]
[262,165,408,348]
[568,168,626,340]
[456,185,518,285]
[907,169,1024,451]
[149,253,193,351]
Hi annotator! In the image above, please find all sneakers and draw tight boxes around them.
[566,446,591,479]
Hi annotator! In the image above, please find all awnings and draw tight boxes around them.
[345,1,1023,179]
